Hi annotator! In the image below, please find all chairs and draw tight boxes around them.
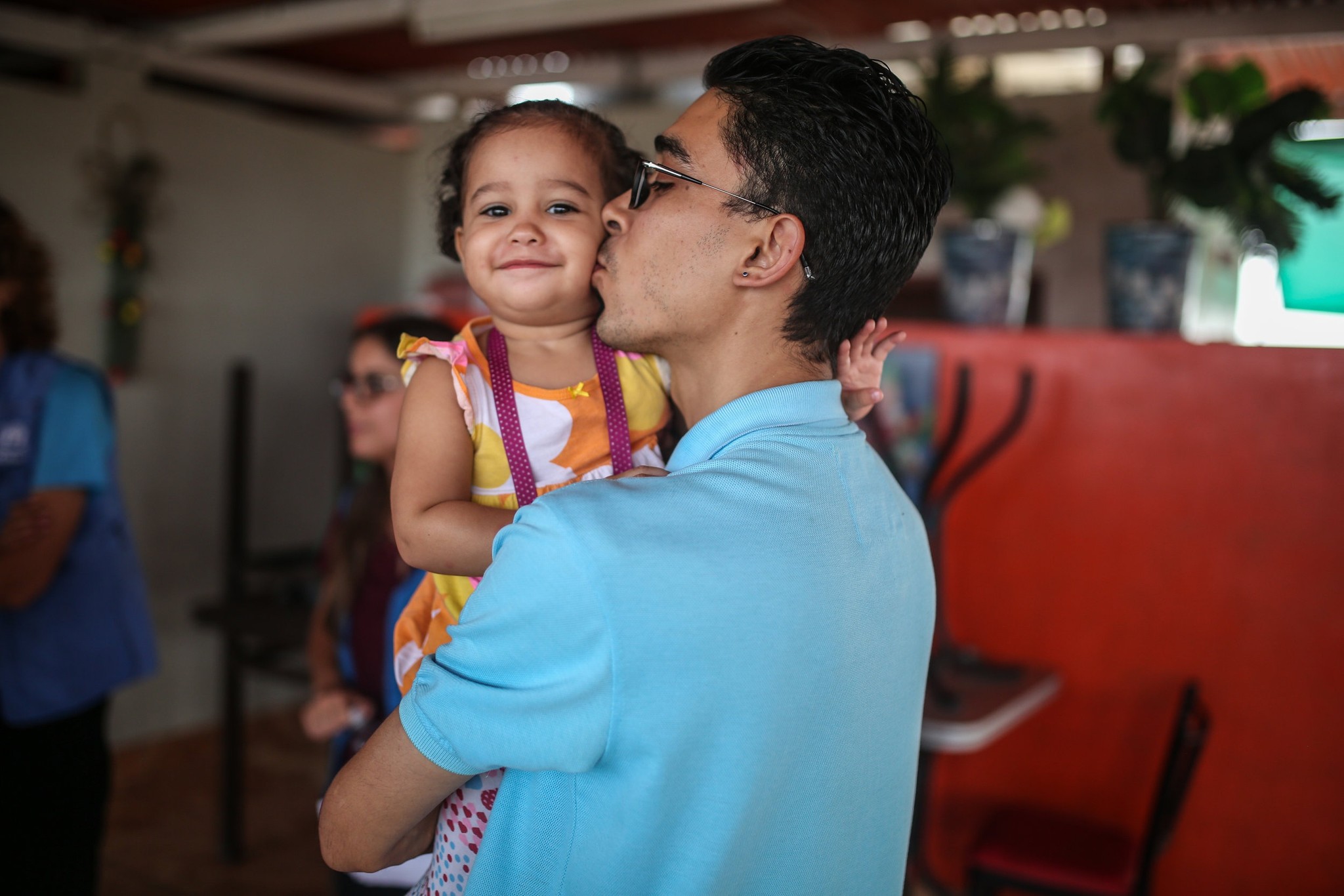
[967,678,1213,896]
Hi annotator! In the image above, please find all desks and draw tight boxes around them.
[920,655,1063,755]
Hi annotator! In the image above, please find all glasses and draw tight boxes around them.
[628,159,814,278]
[339,370,393,398]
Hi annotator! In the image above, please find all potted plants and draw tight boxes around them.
[917,39,1055,326]
[1096,44,1341,336]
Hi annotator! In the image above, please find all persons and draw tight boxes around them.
[386,99,906,896]
[319,34,936,896]
[302,304,460,779]
[1,200,150,895]
